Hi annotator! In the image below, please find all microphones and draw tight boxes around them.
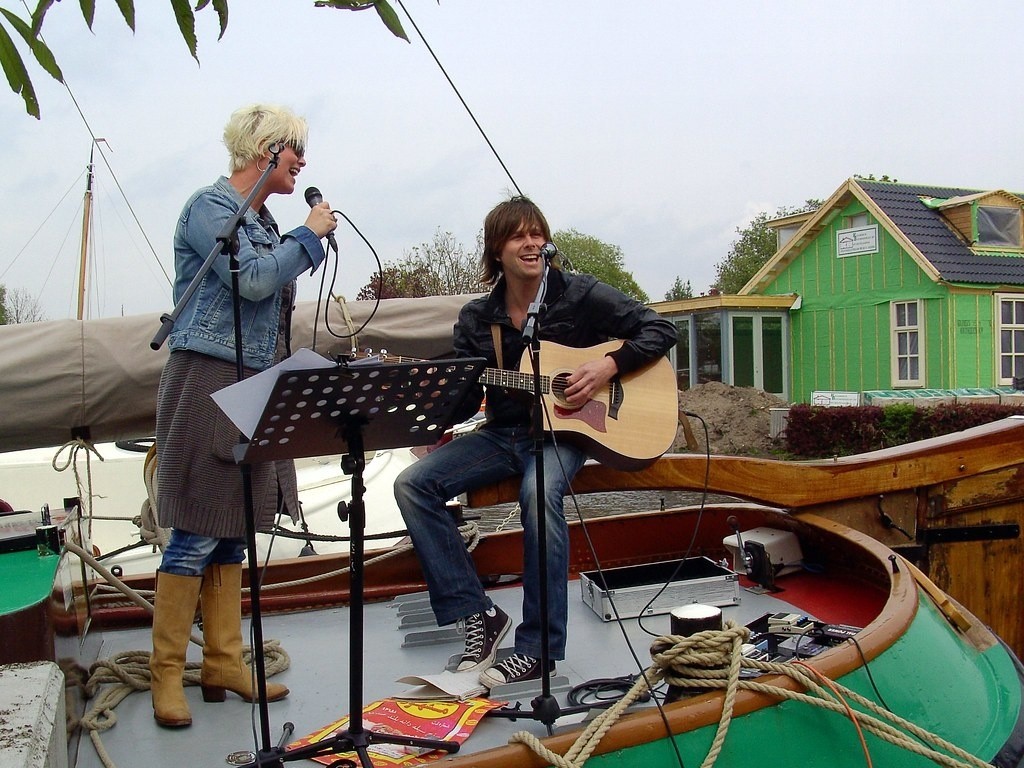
[304,186,339,253]
[539,242,558,259]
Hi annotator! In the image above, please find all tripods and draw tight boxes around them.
[149,142,488,768]
[483,260,651,739]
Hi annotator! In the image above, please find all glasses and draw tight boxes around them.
[277,139,305,158]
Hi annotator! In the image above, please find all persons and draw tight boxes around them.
[391,197,679,692]
[146,102,337,728]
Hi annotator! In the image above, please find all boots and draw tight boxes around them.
[149,566,203,727]
[199,563,290,703]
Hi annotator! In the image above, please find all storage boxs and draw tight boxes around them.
[862,387,1024,409]
[579,555,742,622]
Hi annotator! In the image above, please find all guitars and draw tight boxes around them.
[325,329,682,478]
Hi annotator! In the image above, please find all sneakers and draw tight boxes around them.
[454,602,512,676]
[479,649,556,690]
